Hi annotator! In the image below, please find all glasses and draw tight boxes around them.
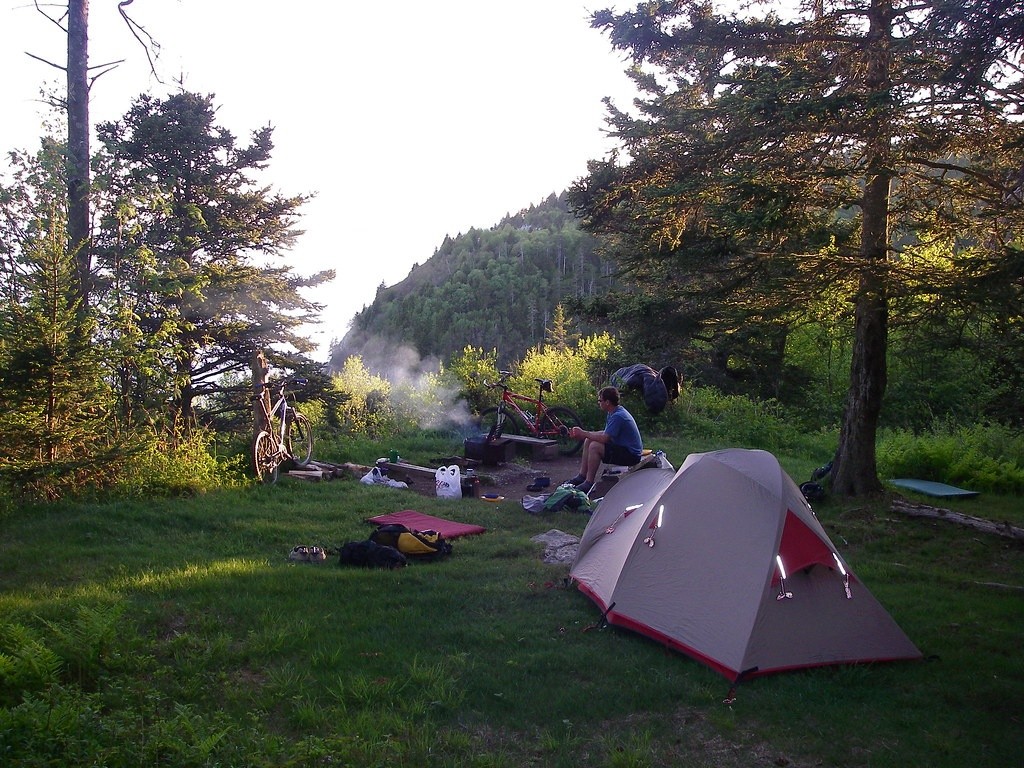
[597,400,606,402]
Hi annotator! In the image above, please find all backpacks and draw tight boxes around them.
[542,485,590,513]
[337,540,409,571]
[369,523,452,564]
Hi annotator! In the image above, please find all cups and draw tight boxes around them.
[389,449,398,462]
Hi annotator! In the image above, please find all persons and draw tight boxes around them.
[564,387,643,494]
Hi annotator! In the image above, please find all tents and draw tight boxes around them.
[568,448,923,681]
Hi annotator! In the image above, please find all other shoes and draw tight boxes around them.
[288,545,309,562]
[309,546,327,564]
[565,474,586,486]
[576,480,594,494]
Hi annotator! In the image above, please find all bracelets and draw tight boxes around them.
[585,431,589,438]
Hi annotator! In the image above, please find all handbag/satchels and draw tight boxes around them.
[435,465,462,498]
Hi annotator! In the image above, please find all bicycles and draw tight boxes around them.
[477,371,585,455]
[249,378,313,487]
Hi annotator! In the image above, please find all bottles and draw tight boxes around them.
[461,468,480,499]
